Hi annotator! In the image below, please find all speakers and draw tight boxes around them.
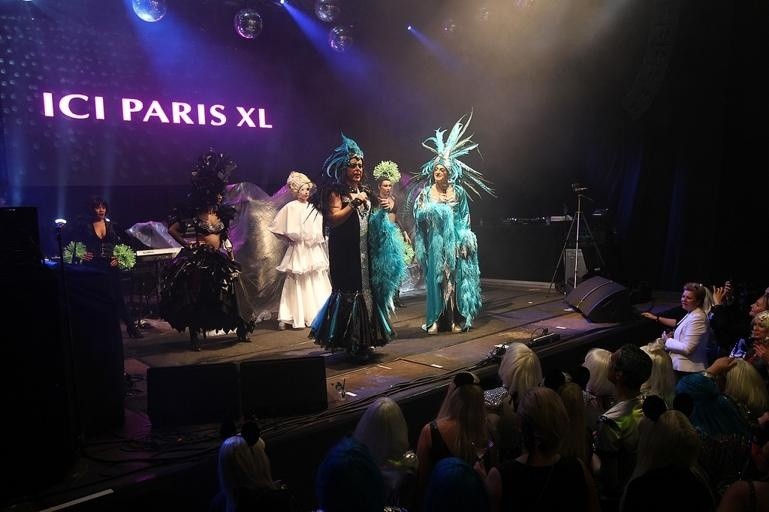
[146,362,240,429]
[579,282,628,323]
[566,275,612,308]
[240,356,328,420]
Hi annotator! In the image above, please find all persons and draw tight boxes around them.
[316,395,418,512]
[367,156,414,311]
[303,135,394,362]
[400,107,500,336]
[165,153,255,352]
[319,456,388,512]
[271,169,335,331]
[211,418,316,512]
[417,276,768,509]
[80,195,144,339]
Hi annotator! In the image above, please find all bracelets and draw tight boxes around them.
[350,196,361,209]
[188,240,195,249]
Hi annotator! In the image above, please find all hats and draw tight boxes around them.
[287,171,311,196]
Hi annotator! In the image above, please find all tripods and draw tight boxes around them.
[547,211,607,294]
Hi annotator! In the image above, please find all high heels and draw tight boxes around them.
[448,321,462,333]
[237,329,253,342]
[191,337,200,351]
[393,298,406,308]
[126,325,144,338]
[428,321,440,334]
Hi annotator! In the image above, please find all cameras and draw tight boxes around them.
[572,184,592,200]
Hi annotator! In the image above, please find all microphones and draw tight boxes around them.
[360,188,369,210]
[442,183,447,194]
